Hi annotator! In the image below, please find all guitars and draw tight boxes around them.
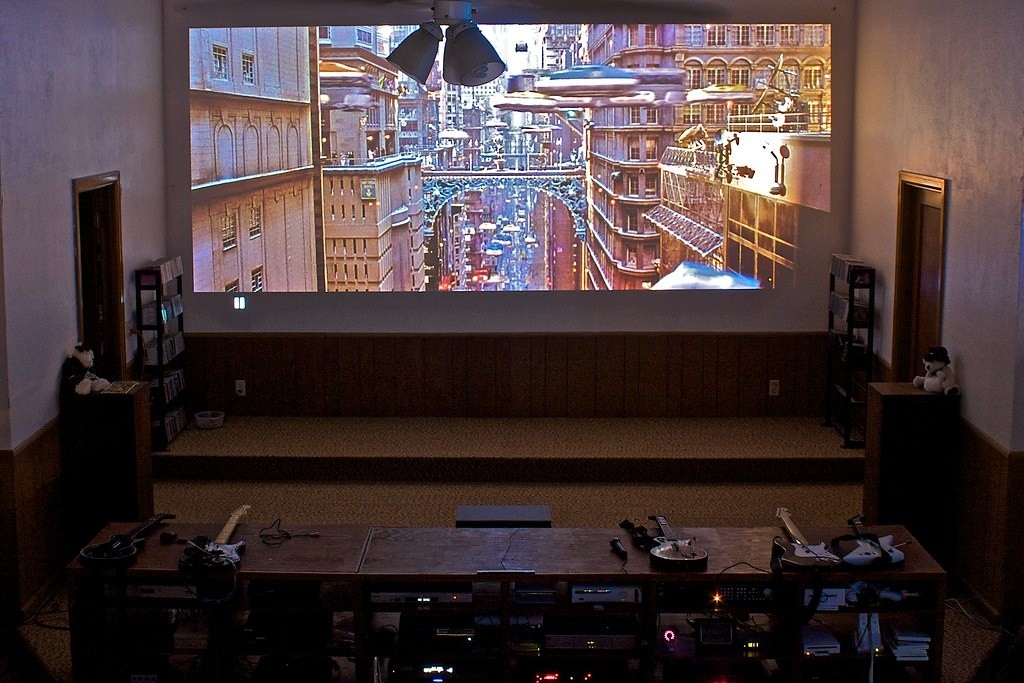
[771,507,842,572]
[647,515,708,573]
[177,503,253,569]
[79,512,175,564]
[841,514,906,569]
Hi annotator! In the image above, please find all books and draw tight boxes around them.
[884,624,931,660]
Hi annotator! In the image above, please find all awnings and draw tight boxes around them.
[641,204,724,257]
[657,146,720,178]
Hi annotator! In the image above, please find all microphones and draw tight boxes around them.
[160,531,189,543]
[611,537,627,557]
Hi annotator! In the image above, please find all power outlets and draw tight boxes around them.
[235,380,246,396]
[769,380,779,396]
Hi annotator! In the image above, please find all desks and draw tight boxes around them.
[68,523,945,683]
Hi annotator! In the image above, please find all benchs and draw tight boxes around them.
[456,506,551,527]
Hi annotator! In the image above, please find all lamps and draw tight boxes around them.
[384,0,506,87]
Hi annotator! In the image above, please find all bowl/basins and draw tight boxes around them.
[194,411,225,430]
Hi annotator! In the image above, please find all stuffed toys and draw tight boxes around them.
[62,343,109,395]
[912,346,961,396]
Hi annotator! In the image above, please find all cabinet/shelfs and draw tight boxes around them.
[135,255,190,452]
[820,253,876,449]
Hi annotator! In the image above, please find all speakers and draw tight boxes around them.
[455,504,552,528]
